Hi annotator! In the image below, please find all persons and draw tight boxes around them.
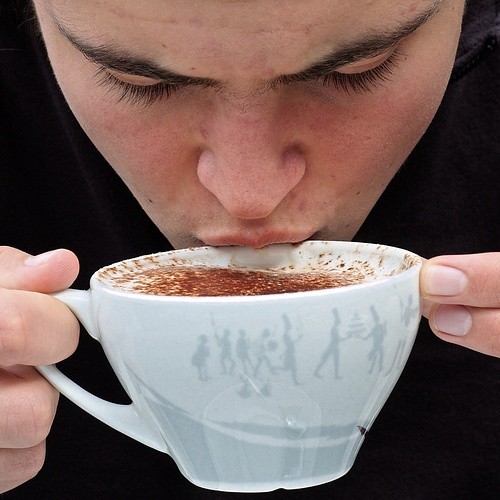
[0,0,500,500]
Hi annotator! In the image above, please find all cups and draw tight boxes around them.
[32,239,427,494]
